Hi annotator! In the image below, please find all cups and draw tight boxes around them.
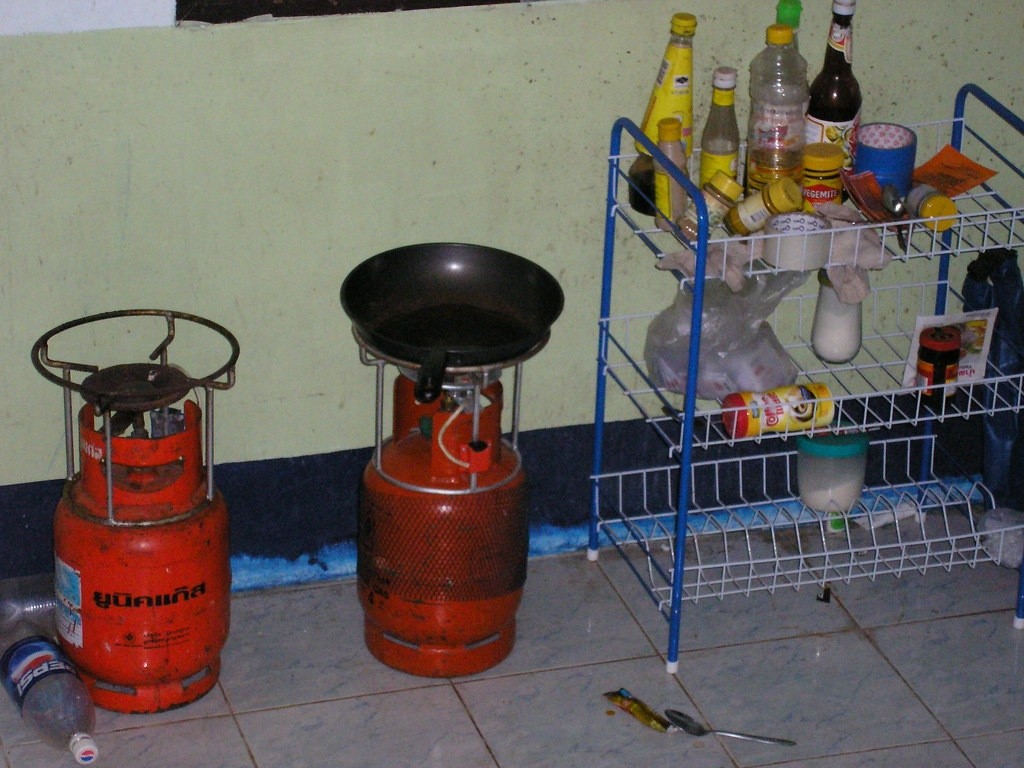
[798,419,870,512]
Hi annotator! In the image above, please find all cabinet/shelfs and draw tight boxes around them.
[586,81,1024,673]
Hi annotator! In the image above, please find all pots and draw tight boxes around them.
[340,242,565,404]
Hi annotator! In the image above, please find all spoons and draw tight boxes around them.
[882,184,907,253]
[664,709,797,747]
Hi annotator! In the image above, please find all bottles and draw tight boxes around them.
[811,268,862,364]
[805,0,863,205]
[776,0,809,117]
[629,12,697,217]
[745,24,810,198]
[655,117,689,232]
[0,618,99,765]
[699,66,740,189]
